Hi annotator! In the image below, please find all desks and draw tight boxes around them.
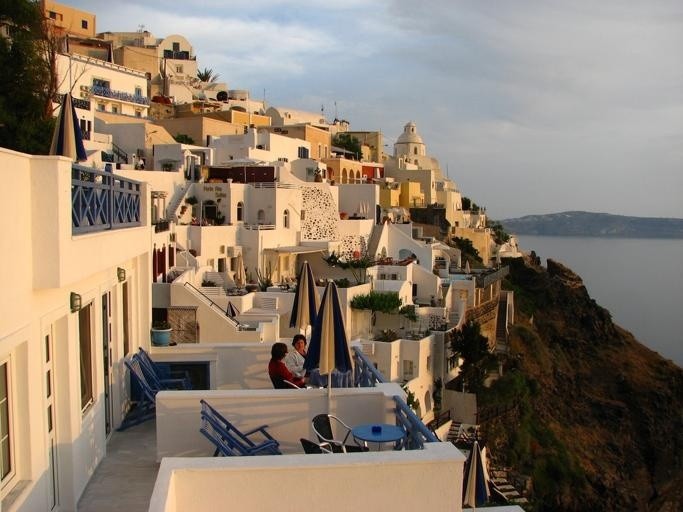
[351,423,406,451]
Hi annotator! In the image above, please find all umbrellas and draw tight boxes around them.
[289,261,321,337]
[463,439,490,512]
[305,279,355,397]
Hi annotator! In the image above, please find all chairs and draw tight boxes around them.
[269,375,300,390]
[131,345,193,390]
[114,353,158,432]
[198,398,370,456]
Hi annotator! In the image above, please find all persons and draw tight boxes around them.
[269,342,307,387]
[285,334,315,375]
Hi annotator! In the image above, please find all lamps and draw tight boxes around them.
[69,290,81,315]
[116,266,126,283]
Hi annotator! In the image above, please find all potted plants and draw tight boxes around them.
[312,166,322,182]
[150,319,174,347]
